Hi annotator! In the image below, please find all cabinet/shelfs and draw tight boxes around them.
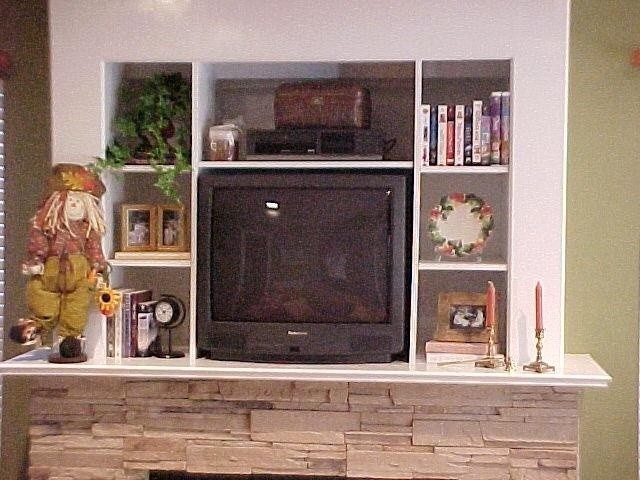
[100,59,515,365]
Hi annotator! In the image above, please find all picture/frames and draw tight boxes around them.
[120,203,157,252]
[436,291,499,343]
[158,204,188,252]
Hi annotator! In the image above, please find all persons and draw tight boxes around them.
[10,163,121,364]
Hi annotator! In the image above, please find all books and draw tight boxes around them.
[107,288,162,358]
[423,91,509,166]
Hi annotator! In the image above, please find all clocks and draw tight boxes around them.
[153,295,186,359]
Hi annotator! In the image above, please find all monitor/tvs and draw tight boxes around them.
[197,173,404,363]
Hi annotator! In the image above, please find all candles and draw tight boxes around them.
[535,280,543,330]
[485,280,495,326]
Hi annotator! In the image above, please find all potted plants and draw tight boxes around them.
[82,71,192,197]
